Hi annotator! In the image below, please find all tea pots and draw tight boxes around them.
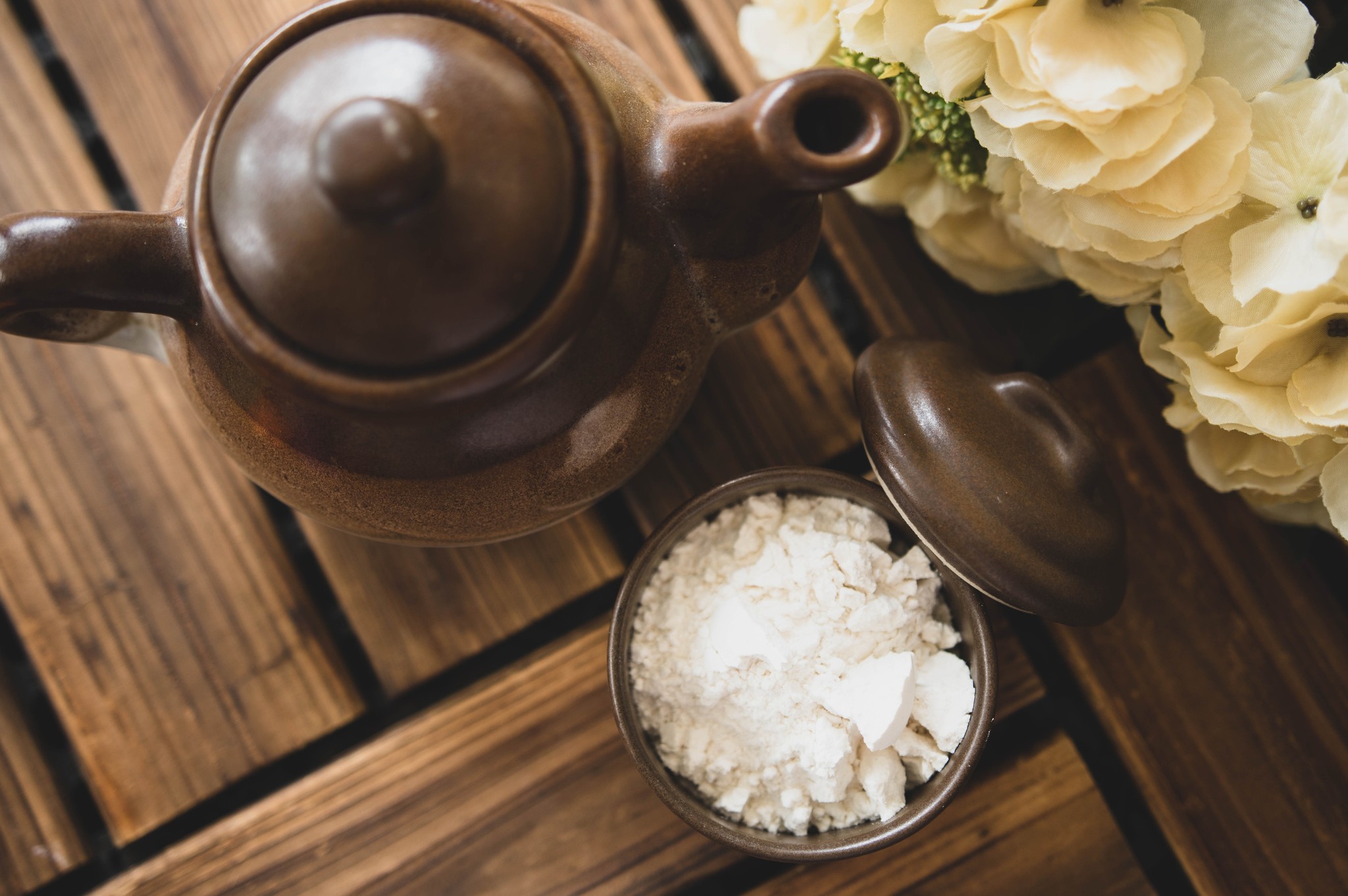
[1,0,906,549]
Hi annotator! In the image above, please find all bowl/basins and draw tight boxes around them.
[605,469,996,865]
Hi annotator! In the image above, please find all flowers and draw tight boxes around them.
[740,0,1348,534]
